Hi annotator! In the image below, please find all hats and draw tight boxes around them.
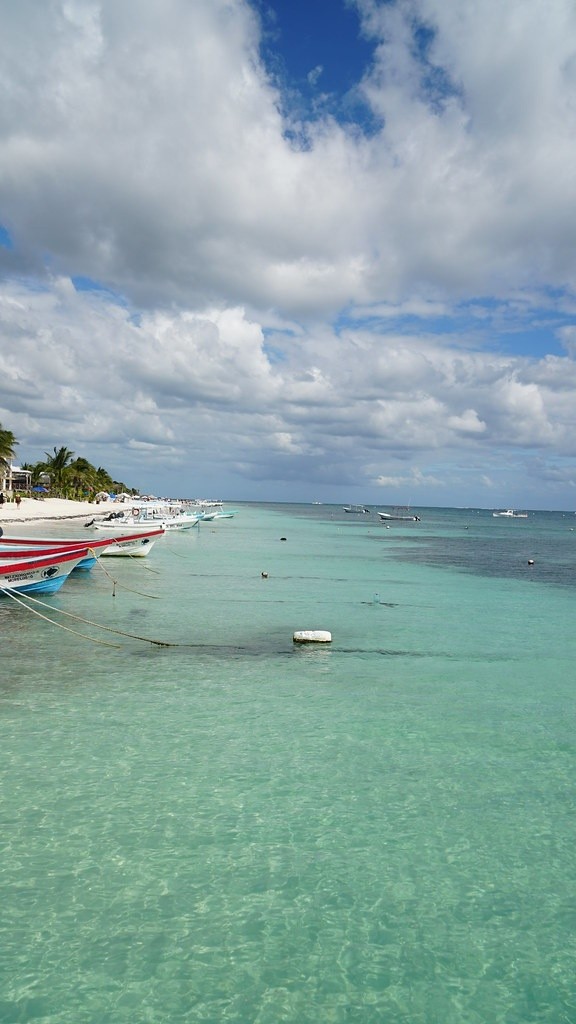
[15,494,21,497]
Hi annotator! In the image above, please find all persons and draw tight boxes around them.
[113,497,124,503]
[96,495,107,504]
[0,493,5,509]
[34,495,44,501]
[15,494,21,510]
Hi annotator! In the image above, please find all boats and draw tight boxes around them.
[312,501,322,505]
[0,499,234,596]
[492,510,528,518]
[377,511,420,521]
[343,504,367,513]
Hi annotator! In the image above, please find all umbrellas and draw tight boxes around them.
[96,492,165,500]
[31,486,49,501]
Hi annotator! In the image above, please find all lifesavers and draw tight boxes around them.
[132,508,140,517]
